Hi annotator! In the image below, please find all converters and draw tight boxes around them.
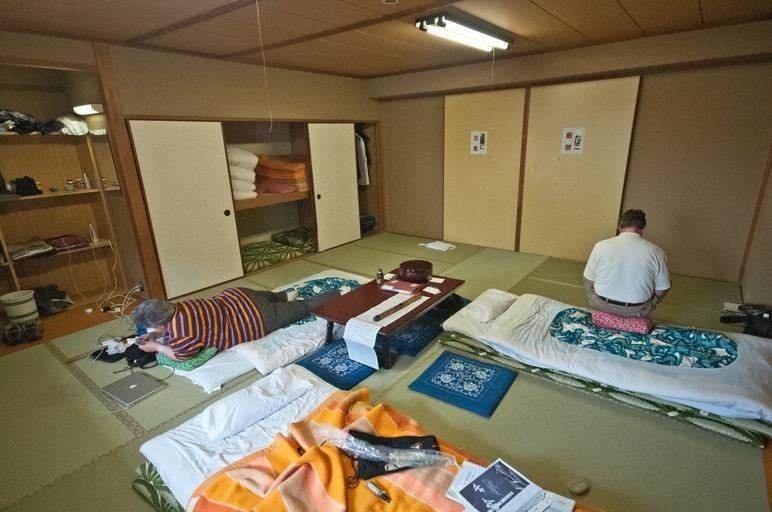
[85,309,93,313]
[103,306,110,312]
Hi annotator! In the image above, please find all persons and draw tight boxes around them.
[583,208,673,318]
[133,284,355,361]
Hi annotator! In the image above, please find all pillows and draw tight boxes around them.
[462,288,519,325]
[198,367,315,444]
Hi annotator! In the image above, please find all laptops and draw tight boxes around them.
[101,372,167,408]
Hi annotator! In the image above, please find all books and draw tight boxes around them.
[444,457,576,512]
[380,277,425,297]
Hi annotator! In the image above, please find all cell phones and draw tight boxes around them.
[135,338,144,345]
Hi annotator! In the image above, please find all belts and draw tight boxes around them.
[595,293,648,308]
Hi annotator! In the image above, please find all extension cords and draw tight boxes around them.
[100,306,121,313]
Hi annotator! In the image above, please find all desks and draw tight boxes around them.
[308,267,467,370]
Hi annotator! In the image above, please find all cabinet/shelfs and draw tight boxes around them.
[0,129,129,320]
[124,111,385,301]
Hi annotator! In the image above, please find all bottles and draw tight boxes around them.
[376,268,384,284]
